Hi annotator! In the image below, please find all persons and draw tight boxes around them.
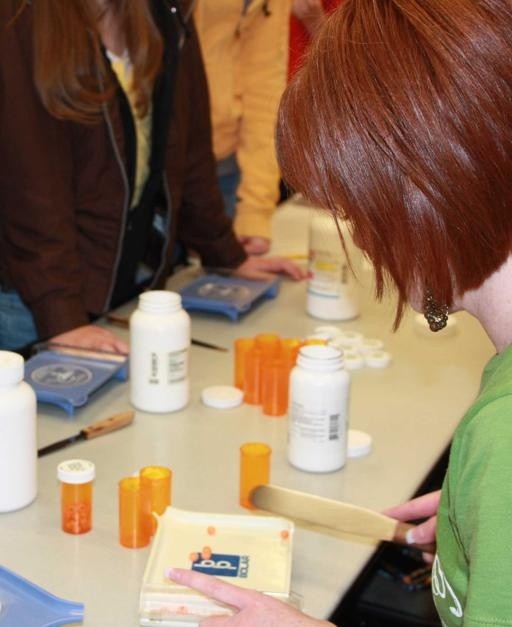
[1,1,311,355]
[154,0,512,627]
[194,0,288,255]
[286,0,351,84]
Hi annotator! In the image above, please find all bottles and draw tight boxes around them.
[0,349,38,512]
[305,201,364,319]
[239,442,272,509]
[287,345,351,472]
[129,290,191,414]
[57,458,95,536]
[118,465,172,549]
[235,332,328,416]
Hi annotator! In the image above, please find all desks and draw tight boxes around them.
[0,196,494,627]
